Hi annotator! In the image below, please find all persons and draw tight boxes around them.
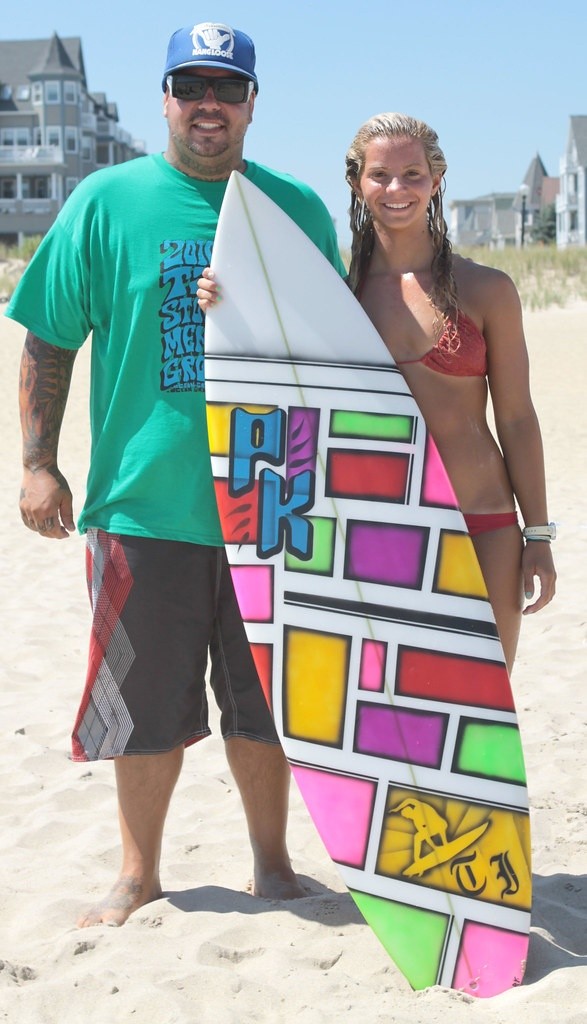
[3,23,349,929]
[196,112,557,682]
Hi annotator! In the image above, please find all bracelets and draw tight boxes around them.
[525,535,551,543]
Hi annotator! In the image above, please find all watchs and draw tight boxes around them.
[523,521,556,540]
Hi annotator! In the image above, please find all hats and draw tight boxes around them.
[162,24,260,96]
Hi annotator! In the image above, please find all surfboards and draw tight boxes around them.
[202,167,533,996]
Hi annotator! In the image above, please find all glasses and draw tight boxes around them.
[167,73,255,105]
